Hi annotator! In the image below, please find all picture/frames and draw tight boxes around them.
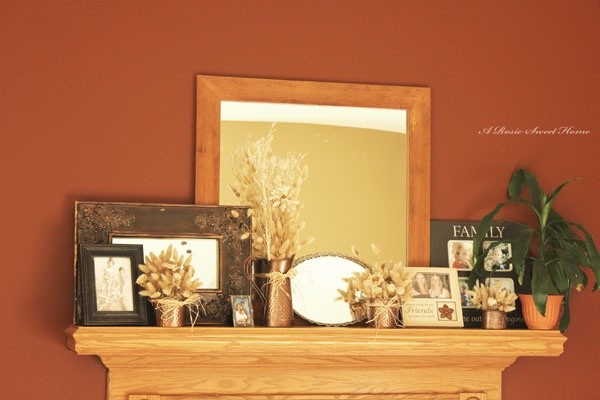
[73,200,253,326]
[227,293,254,327]
[77,243,151,326]
[399,266,465,328]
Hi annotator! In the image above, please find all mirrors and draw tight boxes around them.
[193,73,433,266]
[291,251,377,326]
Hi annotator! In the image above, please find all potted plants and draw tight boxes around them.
[467,168,600,332]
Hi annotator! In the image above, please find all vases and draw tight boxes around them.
[370,299,400,328]
[254,260,296,327]
[149,302,186,327]
[480,309,507,329]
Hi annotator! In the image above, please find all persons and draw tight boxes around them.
[429,275,450,298]
[453,242,471,269]
[410,273,429,299]
[236,302,248,324]
[102,258,115,303]
[493,249,503,269]
[117,266,127,299]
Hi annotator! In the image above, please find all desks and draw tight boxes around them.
[63,325,568,399]
